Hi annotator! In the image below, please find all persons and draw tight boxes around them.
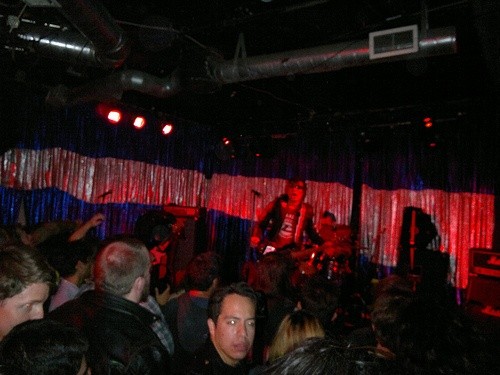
[251,174,323,258]
[1,213,500,375]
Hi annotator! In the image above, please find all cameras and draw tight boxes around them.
[146,264,170,296]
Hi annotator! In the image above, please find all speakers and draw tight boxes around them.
[163,206,207,218]
[468,247,500,278]
[466,277,500,314]
[400,206,439,247]
[167,218,209,269]
[395,247,455,300]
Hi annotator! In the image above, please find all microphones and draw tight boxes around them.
[97,190,112,199]
[251,188,262,198]
